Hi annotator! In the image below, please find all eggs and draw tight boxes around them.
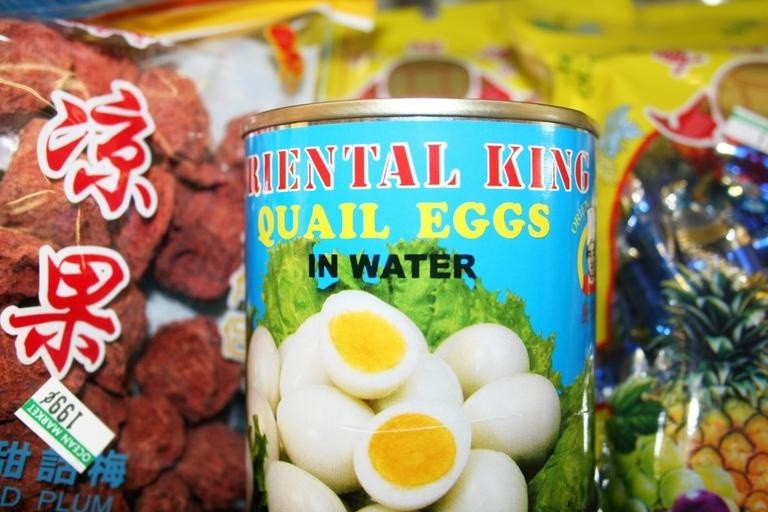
[245,290,563,512]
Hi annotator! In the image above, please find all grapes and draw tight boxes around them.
[595,434,736,512]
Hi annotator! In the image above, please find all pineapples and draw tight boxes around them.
[664,260,768,512]
